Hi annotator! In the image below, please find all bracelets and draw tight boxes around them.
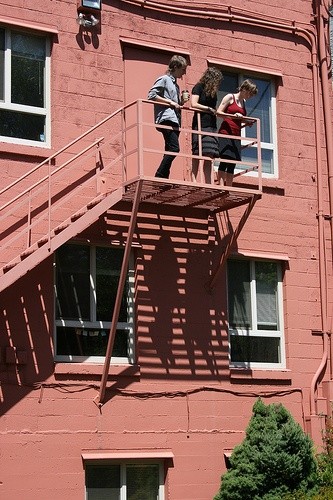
[207,106,211,110]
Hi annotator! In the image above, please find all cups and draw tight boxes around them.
[182,90,189,102]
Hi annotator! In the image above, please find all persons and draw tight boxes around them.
[216,78,258,187]
[190,66,224,185]
[148,55,191,179]
